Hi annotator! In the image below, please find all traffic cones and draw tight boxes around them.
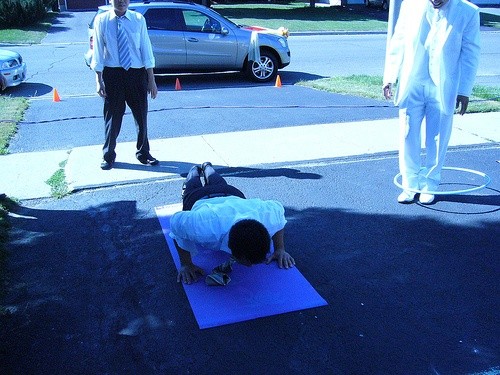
[52,89,61,101]
[174,78,182,90]
[274,75,282,87]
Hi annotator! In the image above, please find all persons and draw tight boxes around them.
[168,162,296,285]
[382,0,481,204]
[90,0,159,169]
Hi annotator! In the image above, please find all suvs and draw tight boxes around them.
[85,0,291,82]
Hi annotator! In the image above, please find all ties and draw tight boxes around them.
[205,256,238,287]
[116,16,133,71]
[428,9,442,86]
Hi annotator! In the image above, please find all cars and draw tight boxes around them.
[0,49,27,92]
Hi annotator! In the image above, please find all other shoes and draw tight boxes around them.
[419,193,435,204]
[147,154,159,165]
[202,162,212,172]
[101,159,111,169]
[398,191,417,203]
